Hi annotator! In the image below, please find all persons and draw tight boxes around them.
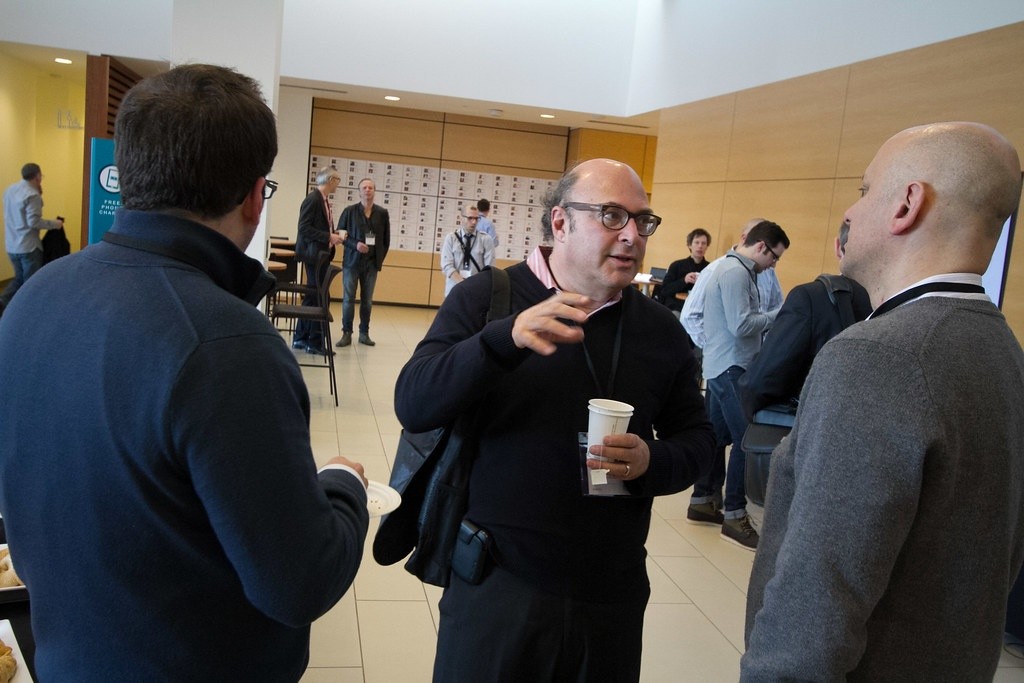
[393,158,719,683]
[441,204,495,298]
[0,64,369,683]
[474,198,500,247]
[740,121,1024,683]
[679,219,790,550]
[336,178,390,347]
[661,228,712,318]
[737,222,872,405]
[292,168,348,357]
[0,163,62,314]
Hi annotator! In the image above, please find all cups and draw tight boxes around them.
[588,399,634,463]
[338,230,346,240]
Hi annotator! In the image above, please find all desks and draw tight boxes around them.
[631,280,660,296]
[676,293,688,299]
[268,239,296,270]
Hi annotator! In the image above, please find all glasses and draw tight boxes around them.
[264,178,278,199]
[562,201,661,236]
[331,176,341,184]
[462,215,482,222]
[756,239,779,263]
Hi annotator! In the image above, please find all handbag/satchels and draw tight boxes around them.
[741,421,794,507]
[374,265,509,587]
[40,216,71,262]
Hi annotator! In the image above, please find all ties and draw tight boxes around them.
[325,198,330,222]
[463,234,473,269]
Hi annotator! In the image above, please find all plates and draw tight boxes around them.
[366,480,402,517]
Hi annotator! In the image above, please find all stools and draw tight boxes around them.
[271,250,343,406]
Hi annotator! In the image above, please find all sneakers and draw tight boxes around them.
[720,514,759,551]
[687,500,724,526]
[336,332,351,346]
[358,332,375,346]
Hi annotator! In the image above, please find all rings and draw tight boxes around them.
[623,465,630,476]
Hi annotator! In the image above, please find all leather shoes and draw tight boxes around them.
[292,340,309,348]
[306,345,336,356]
